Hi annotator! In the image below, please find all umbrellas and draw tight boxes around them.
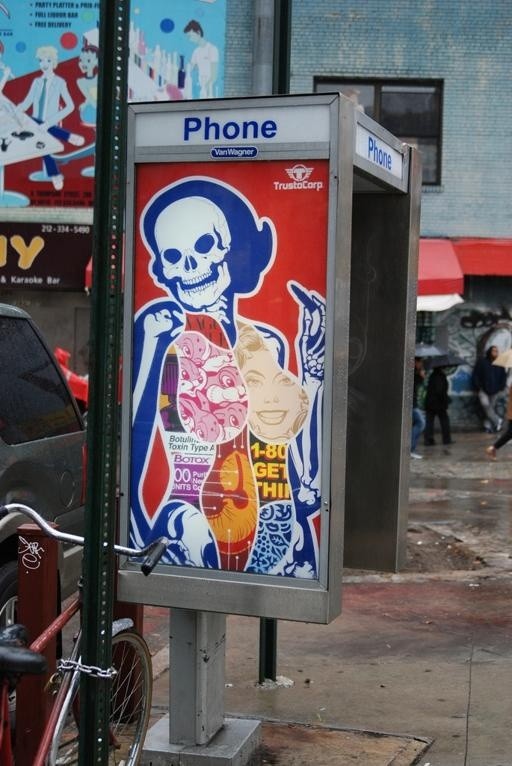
[414,341,449,358]
[490,349,512,370]
[425,355,469,368]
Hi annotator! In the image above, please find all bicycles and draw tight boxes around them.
[0,503,171,766]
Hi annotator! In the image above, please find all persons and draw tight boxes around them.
[410,355,426,459]
[232,325,310,443]
[484,379,512,461]
[472,344,506,434]
[424,367,456,446]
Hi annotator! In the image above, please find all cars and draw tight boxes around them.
[0,304,87,693]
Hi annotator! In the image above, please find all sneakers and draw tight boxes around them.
[485,445,497,462]
[409,451,424,460]
